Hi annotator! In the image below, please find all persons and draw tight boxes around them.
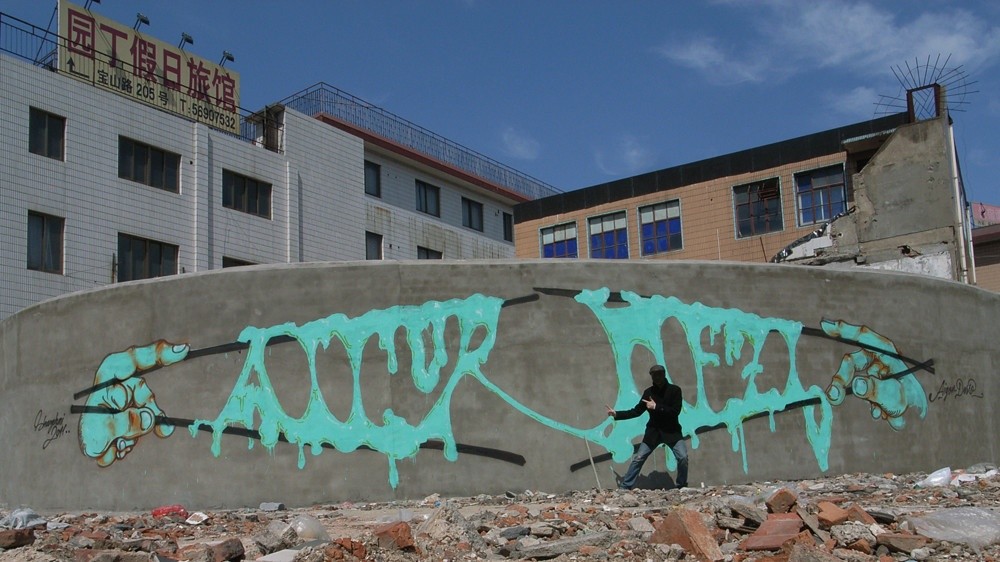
[605,365,688,490]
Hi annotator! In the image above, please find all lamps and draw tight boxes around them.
[178,33,193,48]
[218,50,234,67]
[84,0,101,10]
[133,12,150,31]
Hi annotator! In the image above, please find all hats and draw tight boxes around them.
[649,365,665,375]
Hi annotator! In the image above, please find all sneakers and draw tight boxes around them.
[619,483,631,490]
